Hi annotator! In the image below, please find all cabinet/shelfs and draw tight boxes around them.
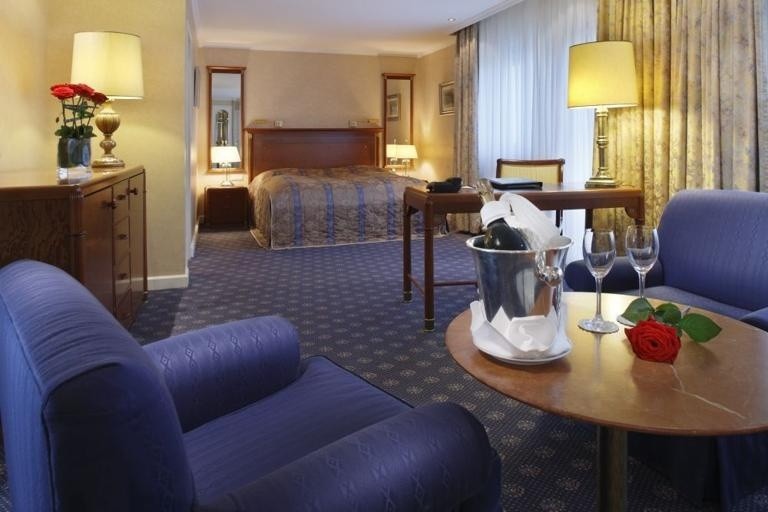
[4,165,149,330]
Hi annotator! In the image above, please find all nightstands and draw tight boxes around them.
[206,187,249,230]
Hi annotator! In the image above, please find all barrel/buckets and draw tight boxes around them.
[465,235,573,324]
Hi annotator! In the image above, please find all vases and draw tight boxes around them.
[57,138,93,186]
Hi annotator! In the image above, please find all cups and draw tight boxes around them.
[577,227,621,335]
[617,226,660,327]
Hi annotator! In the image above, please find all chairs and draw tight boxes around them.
[496,158,566,233]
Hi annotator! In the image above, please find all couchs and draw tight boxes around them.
[566,190,768,509]
[0,258,499,510]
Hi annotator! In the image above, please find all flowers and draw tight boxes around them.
[49,84,107,167]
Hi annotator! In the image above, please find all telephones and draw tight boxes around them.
[426,177,462,193]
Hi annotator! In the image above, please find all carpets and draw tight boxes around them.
[129,224,727,508]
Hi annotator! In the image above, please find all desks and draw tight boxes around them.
[403,185,648,332]
[448,292,768,512]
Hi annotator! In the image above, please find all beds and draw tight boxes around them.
[247,126,459,250]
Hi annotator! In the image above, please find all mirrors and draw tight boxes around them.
[382,72,413,171]
[207,65,246,175]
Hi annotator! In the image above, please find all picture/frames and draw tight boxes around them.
[438,79,455,117]
[387,94,400,121]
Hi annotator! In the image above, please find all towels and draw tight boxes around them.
[471,301,571,359]
[498,194,566,251]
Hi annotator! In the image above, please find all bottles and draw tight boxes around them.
[475,178,542,322]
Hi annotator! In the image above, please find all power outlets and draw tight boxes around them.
[349,120,357,126]
[274,120,283,126]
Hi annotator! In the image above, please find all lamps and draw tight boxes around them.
[211,146,241,187]
[395,144,417,177]
[70,30,145,167]
[386,143,398,164]
[569,41,638,190]
